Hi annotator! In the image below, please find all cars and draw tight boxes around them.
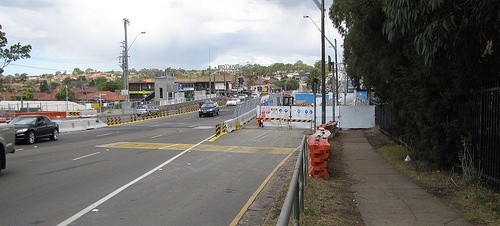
[6,115,59,144]
[226,98,241,106]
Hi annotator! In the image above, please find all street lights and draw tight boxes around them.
[122,31,145,104]
[302,15,338,106]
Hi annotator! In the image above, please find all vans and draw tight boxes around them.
[136,105,159,116]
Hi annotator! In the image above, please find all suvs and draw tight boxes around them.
[199,101,219,116]
